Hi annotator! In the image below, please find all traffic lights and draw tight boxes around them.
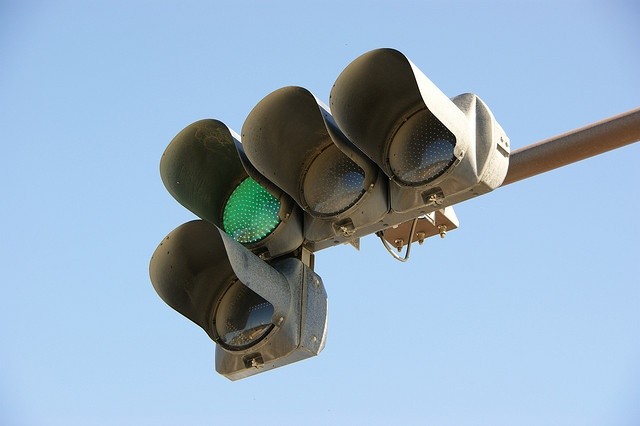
[149,219,328,382]
[159,47,509,260]
